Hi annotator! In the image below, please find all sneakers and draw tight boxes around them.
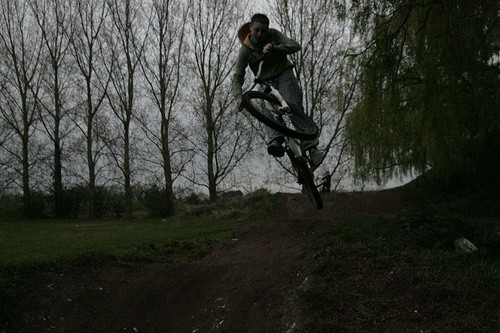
[308,146,322,166]
[268,139,285,157]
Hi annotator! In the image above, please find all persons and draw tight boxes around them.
[232,14,325,167]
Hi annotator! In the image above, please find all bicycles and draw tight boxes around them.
[242,50,324,210]
